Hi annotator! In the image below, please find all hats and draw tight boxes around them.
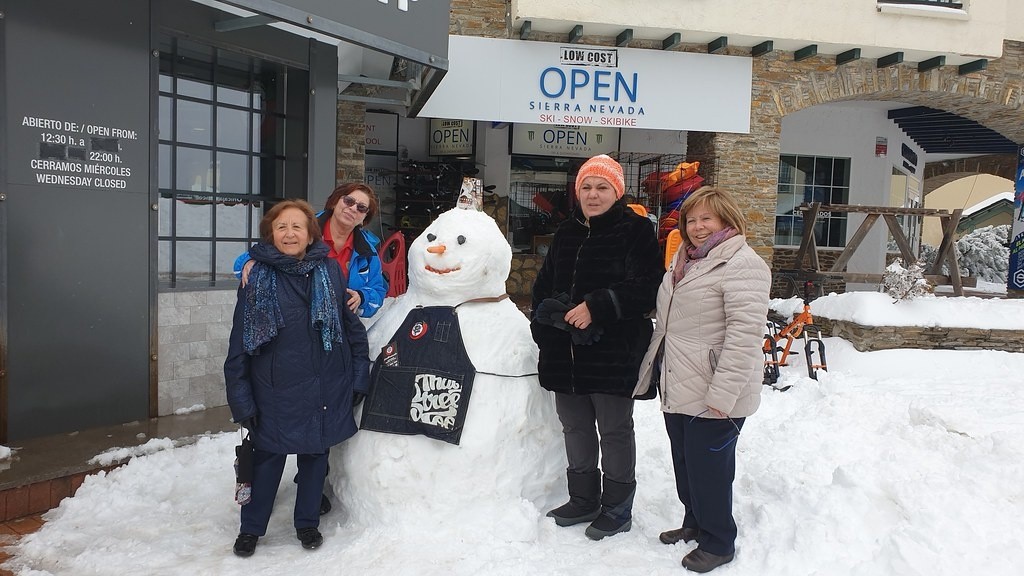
[575,154,625,200]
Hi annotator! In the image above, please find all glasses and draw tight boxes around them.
[343,195,370,214]
[689,408,740,451]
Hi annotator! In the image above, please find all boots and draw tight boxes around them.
[585,474,636,541]
[546,467,602,527]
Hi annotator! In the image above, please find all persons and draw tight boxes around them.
[632,184,772,573]
[222,181,390,559]
[526,151,665,542]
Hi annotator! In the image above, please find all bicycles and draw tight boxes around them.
[761,279,827,391]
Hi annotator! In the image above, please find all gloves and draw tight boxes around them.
[353,392,365,407]
[240,416,258,435]
[536,292,604,345]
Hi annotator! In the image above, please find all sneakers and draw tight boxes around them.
[660,526,699,545]
[233,533,257,556]
[682,546,734,573]
[320,493,331,515]
[297,526,323,549]
